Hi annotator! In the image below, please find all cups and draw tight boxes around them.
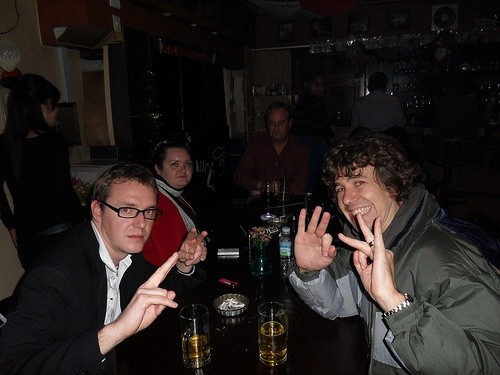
[179,303,212,369]
[257,301,287,365]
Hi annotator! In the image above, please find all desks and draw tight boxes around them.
[107,194,370,375]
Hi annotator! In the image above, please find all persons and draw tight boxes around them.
[287,127,500,375]
[350,72,404,132]
[141,139,211,266]
[0,74,85,272]
[0,161,208,375]
[233,101,310,197]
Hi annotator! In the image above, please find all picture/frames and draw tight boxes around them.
[348,13,369,34]
[277,19,295,44]
[309,16,333,38]
[386,10,412,32]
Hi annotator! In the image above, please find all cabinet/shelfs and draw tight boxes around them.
[312,42,500,131]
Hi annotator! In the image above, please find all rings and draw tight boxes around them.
[369,240,374,247]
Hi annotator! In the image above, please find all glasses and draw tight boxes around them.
[98,200,164,220]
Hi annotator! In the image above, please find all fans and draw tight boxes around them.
[430,3,459,30]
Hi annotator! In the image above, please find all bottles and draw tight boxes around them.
[279,227,294,275]
[250,79,287,97]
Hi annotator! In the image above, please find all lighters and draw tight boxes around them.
[219,278,239,286]
